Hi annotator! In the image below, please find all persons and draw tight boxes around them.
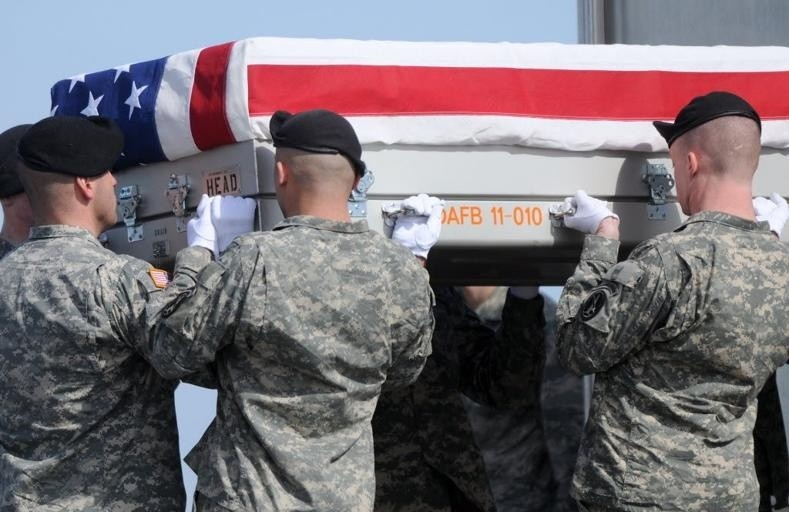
[153,111,444,512]
[372,282,583,512]
[0,117,190,512]
[556,91,789,512]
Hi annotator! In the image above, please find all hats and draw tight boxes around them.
[0,123,34,199]
[269,108,365,179]
[17,113,126,177]
[652,91,761,148]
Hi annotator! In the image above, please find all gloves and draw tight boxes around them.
[210,195,257,253]
[563,189,619,235]
[187,193,221,262]
[391,192,446,261]
[752,190,789,240]
